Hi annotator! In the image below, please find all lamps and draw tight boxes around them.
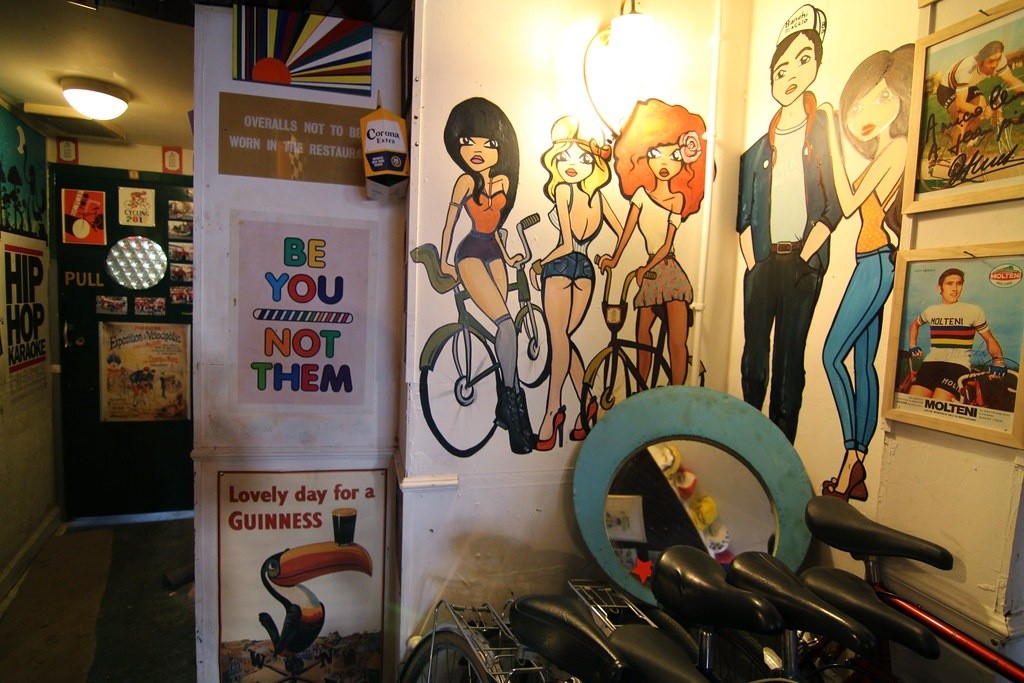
[58,76,130,122]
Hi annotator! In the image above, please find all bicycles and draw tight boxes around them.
[397,493,1022,683]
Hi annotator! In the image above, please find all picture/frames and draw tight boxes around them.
[162,146,183,174]
[899,0,1024,215]
[56,137,79,164]
[882,240,1024,450]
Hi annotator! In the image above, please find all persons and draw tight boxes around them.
[908,268,1007,402]
[937,40,1024,171]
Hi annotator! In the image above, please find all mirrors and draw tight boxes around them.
[572,385,814,605]
